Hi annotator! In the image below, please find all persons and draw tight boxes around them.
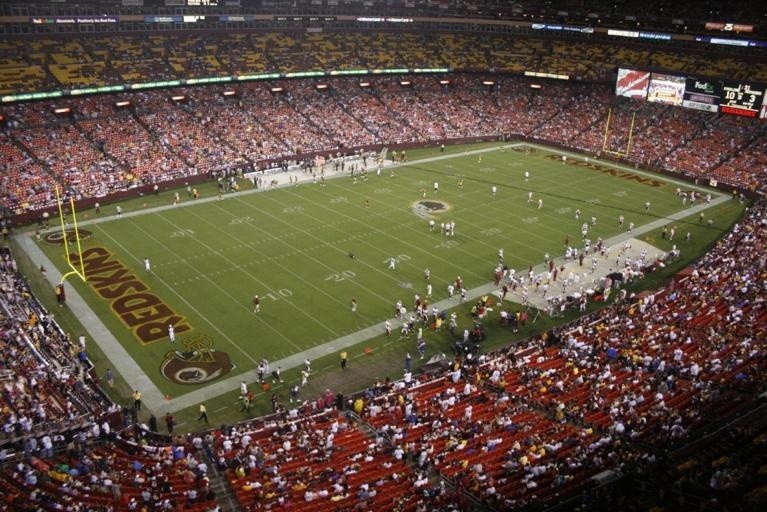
[0,222,766,510]
[1,1,766,238]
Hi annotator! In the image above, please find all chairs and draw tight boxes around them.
[1,26,766,226]
[2,201,767,512]
